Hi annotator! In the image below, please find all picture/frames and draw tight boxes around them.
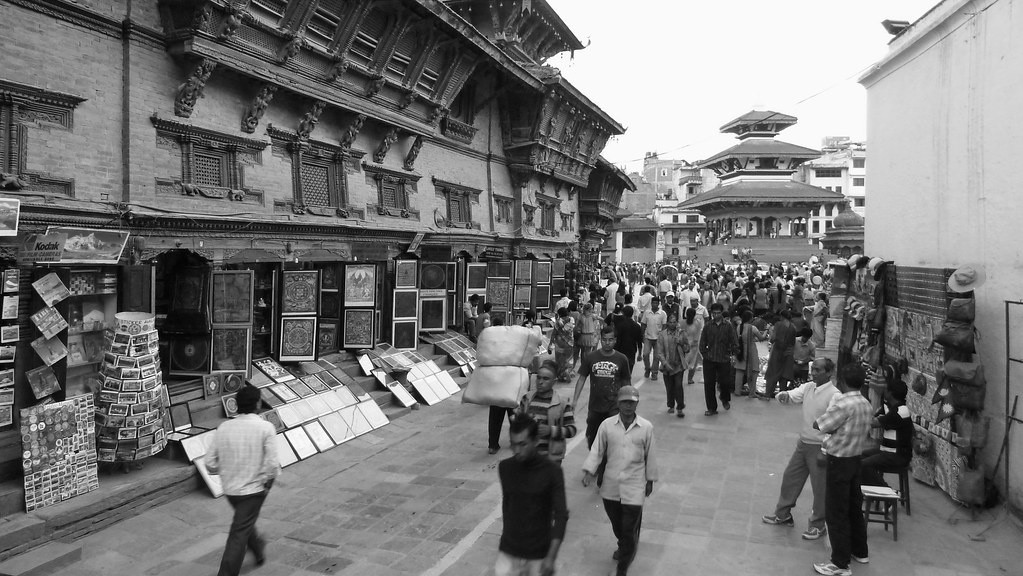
[168,258,566,498]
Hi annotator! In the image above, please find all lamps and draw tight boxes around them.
[883,19,910,34]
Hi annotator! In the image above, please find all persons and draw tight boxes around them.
[493,412,569,576]
[580,386,659,576]
[769,227,776,239]
[695,229,732,247]
[731,246,752,262]
[762,356,912,576]
[462,253,835,455]
[206,386,278,576]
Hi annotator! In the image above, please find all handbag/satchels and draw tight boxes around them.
[931,299,989,508]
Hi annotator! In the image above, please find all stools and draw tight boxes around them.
[860,485,897,542]
[875,468,912,515]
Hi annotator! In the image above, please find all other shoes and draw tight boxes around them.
[644,370,658,380]
[668,408,685,417]
[705,411,718,416]
[720,397,730,410]
[613,546,622,560]
[688,380,694,384]
[559,370,571,383]
[488,445,501,454]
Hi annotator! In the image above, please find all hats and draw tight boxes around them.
[690,296,699,301]
[538,360,558,377]
[882,363,897,384]
[848,253,894,280]
[617,385,639,402]
[913,374,927,395]
[947,265,987,293]
[666,291,675,297]
[844,296,877,322]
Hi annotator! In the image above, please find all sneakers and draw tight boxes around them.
[813,561,852,576]
[851,554,869,564]
[762,514,794,527]
[802,525,826,540]
